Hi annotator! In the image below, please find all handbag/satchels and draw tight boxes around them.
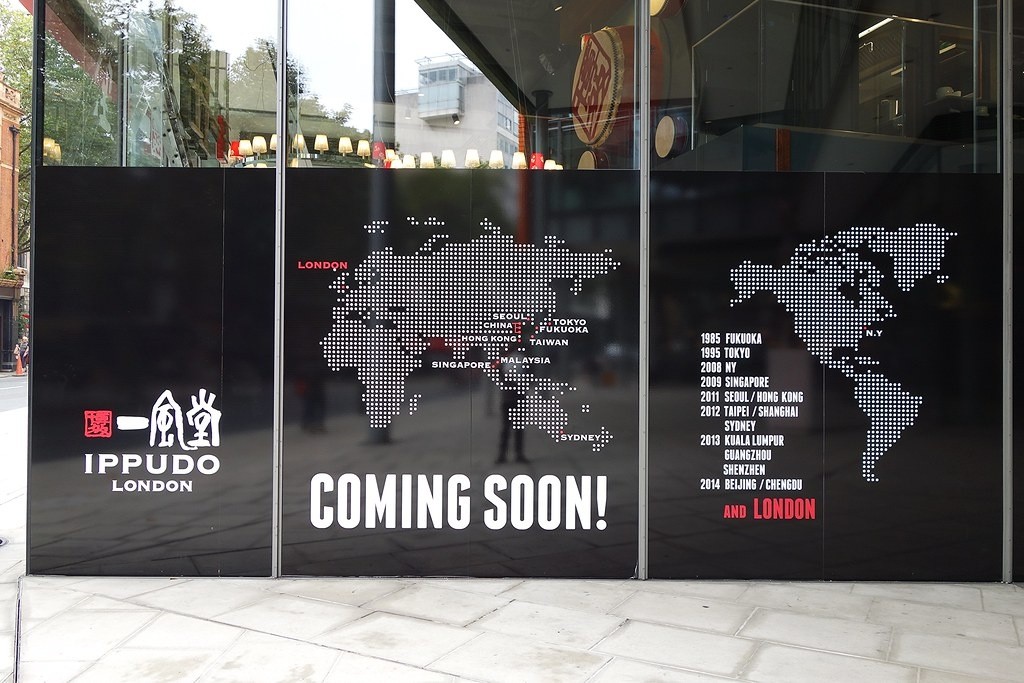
[26,354,29,364]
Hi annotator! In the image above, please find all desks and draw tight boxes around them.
[861,96,1024,143]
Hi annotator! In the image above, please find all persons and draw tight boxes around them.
[493,344,534,464]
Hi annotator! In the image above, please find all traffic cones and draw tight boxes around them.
[11,356,26,377]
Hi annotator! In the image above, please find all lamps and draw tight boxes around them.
[43,136,62,167]
[238,140,254,163]
[293,134,306,153]
[373,142,386,165]
[356,139,371,161]
[314,134,329,155]
[252,135,268,160]
[385,149,416,169]
[338,136,353,157]
[530,153,563,170]
[419,152,435,169]
[451,113,461,125]
[270,133,277,150]
[489,150,505,170]
[464,149,481,170]
[512,152,528,170]
[440,149,456,169]
